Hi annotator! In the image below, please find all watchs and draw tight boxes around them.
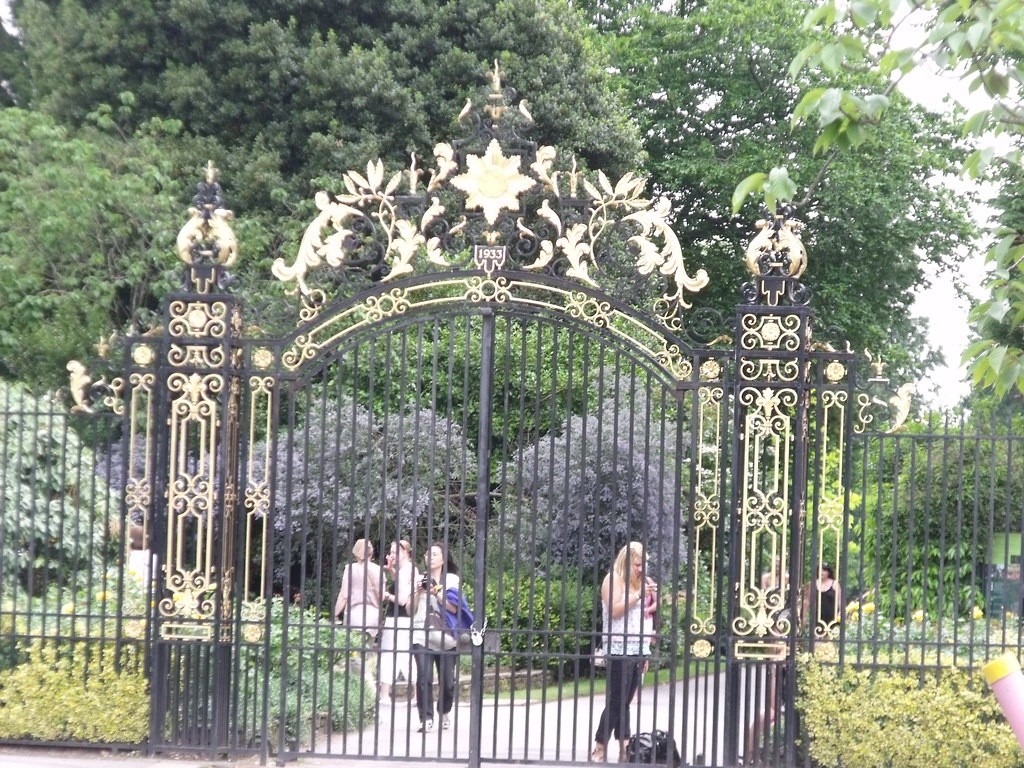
[634,590,641,601]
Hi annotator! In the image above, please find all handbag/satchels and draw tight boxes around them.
[423,605,457,651]
[625,729,681,768]
[590,648,606,667]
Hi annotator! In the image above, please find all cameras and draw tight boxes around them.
[421,571,437,590]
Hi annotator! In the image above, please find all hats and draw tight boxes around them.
[352,539,373,560]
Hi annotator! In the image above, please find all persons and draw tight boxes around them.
[803,562,841,633]
[760,554,790,596]
[589,541,658,764]
[127,527,151,577]
[333,538,462,733]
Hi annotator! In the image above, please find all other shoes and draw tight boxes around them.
[441,714,449,729]
[591,747,605,764]
[404,698,417,706]
[379,697,391,706]
[417,717,432,732]
